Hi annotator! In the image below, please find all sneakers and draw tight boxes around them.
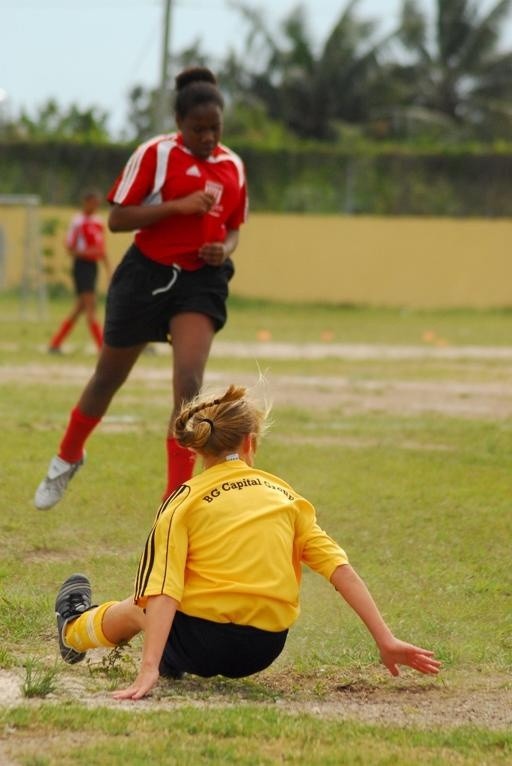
[33,447,87,512]
[47,345,72,361]
[54,573,93,666]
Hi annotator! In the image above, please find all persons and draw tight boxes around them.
[31,63,252,512]
[44,181,111,357]
[54,382,446,701]
